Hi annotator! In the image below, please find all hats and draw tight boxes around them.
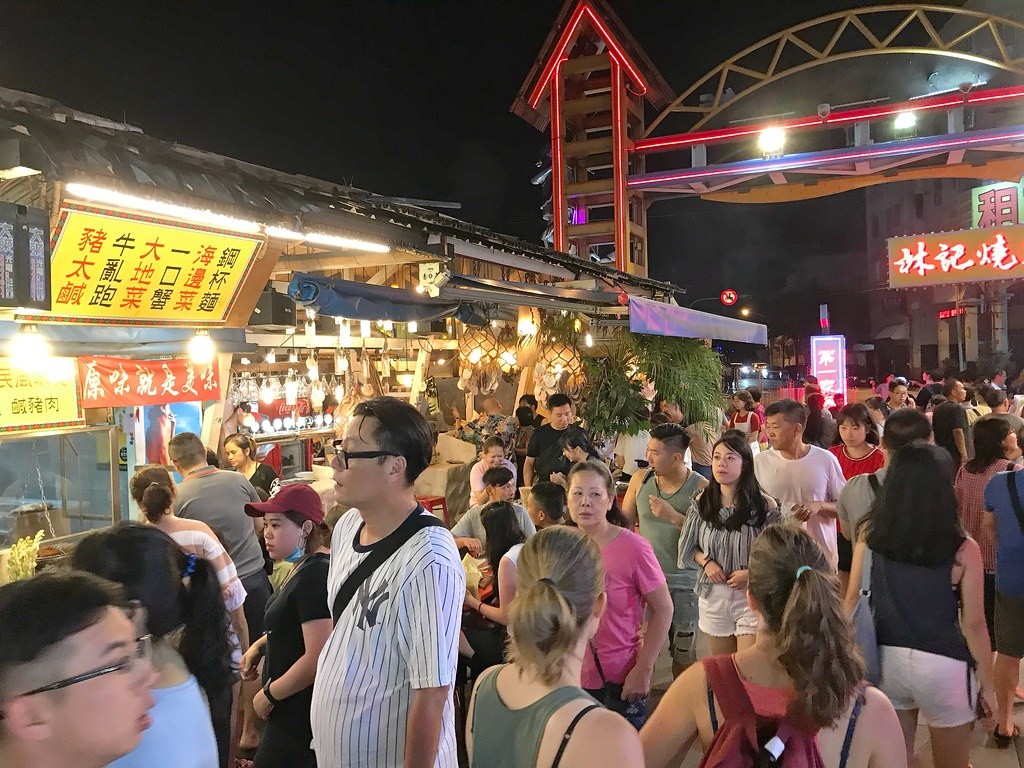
[244,485,324,526]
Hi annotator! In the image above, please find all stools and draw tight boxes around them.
[416,495,451,530]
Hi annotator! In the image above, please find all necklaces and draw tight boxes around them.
[654,468,690,500]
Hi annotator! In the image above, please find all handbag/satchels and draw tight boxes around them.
[604,680,648,727]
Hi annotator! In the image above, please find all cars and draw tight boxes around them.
[738,362,794,381]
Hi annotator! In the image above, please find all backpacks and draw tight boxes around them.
[698,654,873,767]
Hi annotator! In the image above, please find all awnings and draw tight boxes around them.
[443,274,767,346]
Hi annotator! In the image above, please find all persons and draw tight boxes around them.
[0,369,1024,768]
[145,403,176,479]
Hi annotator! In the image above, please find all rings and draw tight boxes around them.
[807,510,810,513]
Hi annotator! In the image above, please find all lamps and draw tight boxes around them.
[434,261,453,287]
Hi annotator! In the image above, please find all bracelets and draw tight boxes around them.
[703,558,713,569]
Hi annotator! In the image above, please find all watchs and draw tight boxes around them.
[263,677,278,704]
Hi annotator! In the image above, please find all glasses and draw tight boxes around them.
[891,392,907,397]
[333,438,410,469]
[0,632,154,722]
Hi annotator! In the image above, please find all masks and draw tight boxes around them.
[284,528,309,562]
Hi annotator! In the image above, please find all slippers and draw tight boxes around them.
[995,720,1020,748]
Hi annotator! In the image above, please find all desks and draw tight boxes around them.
[462,561,495,611]
